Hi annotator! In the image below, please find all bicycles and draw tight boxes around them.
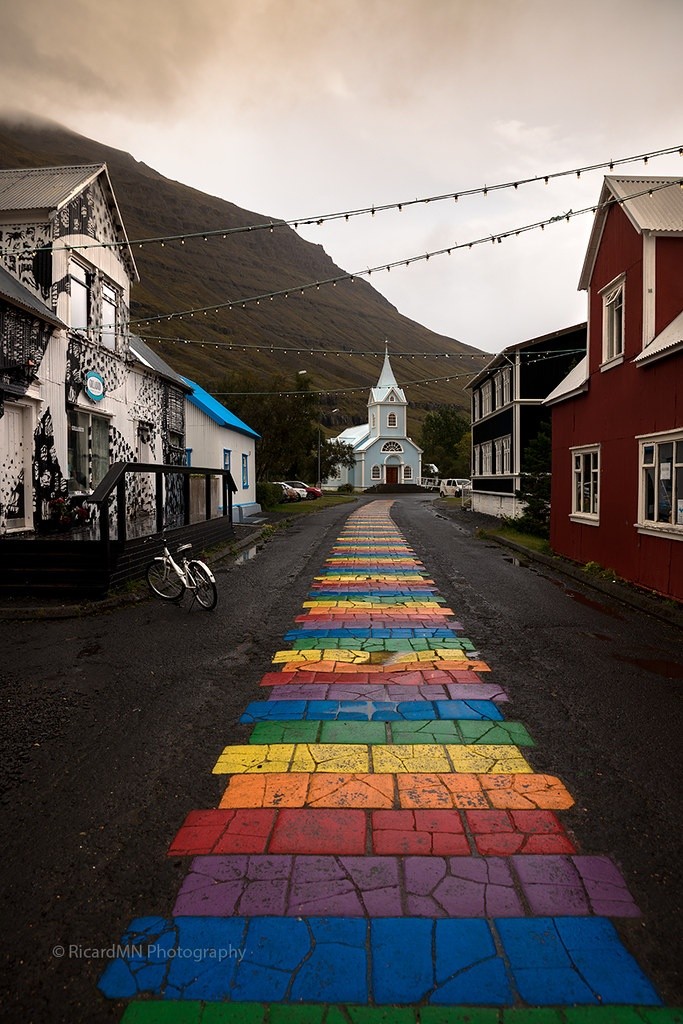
[143,519,218,613]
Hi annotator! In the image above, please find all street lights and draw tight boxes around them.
[317,407,340,487]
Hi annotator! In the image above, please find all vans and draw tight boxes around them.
[440,479,471,498]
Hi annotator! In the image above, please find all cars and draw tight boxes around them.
[284,481,323,500]
[271,481,308,502]
